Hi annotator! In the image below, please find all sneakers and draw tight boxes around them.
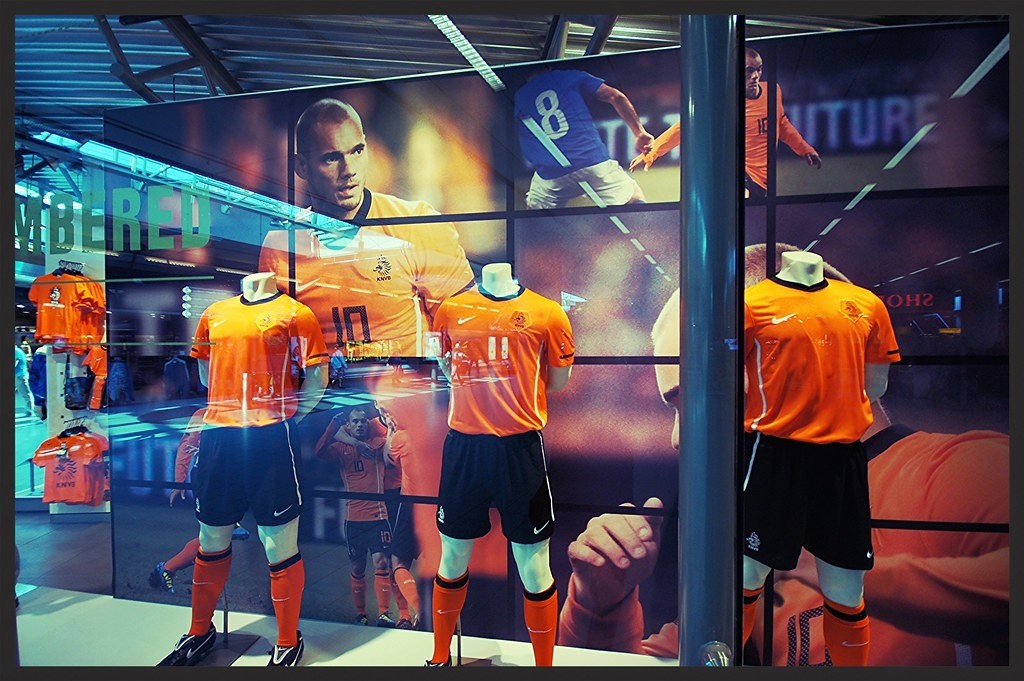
[267,629,304,667]
[396,618,412,630]
[424,655,452,667]
[376,611,396,627]
[351,613,368,626]
[154,560,175,596]
[157,622,217,666]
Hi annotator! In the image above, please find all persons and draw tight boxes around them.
[156,406,249,594]
[739,250,902,666]
[423,263,575,668]
[628,47,823,199]
[315,399,422,630]
[514,62,654,209]
[15,345,35,416]
[556,242,1010,666]
[173,272,329,666]
[257,97,475,355]
[290,346,299,376]
[330,341,349,388]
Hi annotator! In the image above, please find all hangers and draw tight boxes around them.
[52,259,84,277]
[58,417,92,439]
